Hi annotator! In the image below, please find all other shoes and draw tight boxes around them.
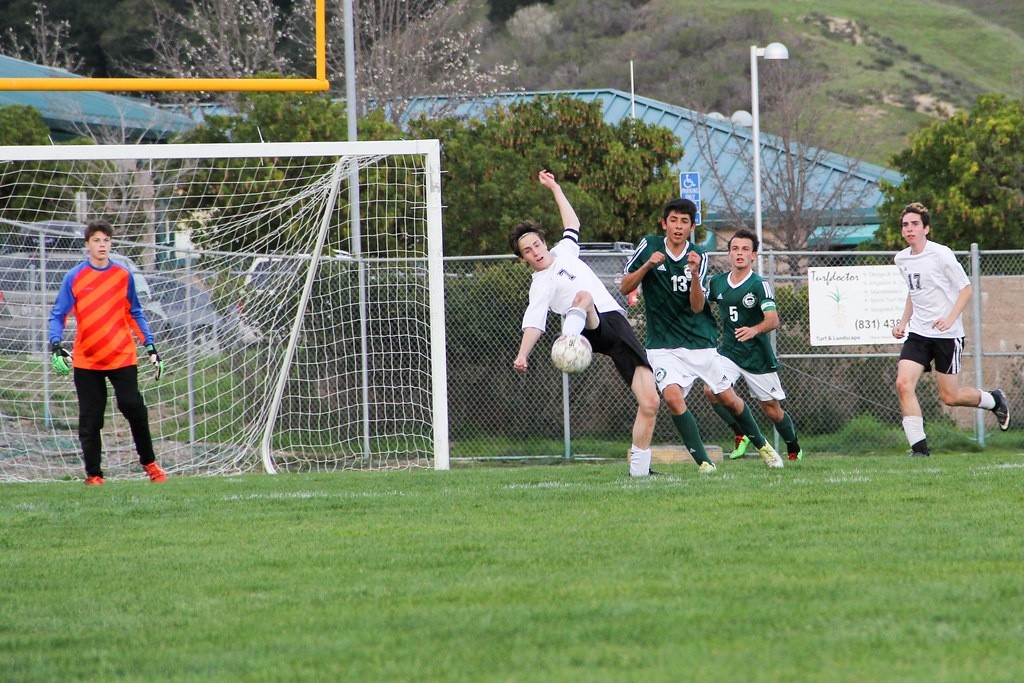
[648,469,662,475]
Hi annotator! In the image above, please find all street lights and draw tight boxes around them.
[745,39,791,455]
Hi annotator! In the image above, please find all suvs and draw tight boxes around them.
[576,240,643,309]
[0,221,155,356]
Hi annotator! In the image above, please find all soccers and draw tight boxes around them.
[551,333,593,373]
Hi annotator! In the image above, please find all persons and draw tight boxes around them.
[892,202,1010,458]
[509,169,803,478]
[48,221,168,486]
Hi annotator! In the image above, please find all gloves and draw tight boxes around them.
[145,343,164,380]
[51,341,73,376]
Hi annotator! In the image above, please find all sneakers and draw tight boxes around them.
[754,438,783,468]
[787,449,803,461]
[990,389,1011,431]
[142,462,168,484]
[699,462,716,474]
[910,448,931,457]
[83,475,105,486]
[729,434,751,459]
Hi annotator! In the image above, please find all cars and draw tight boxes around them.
[142,272,234,357]
[232,255,319,348]
